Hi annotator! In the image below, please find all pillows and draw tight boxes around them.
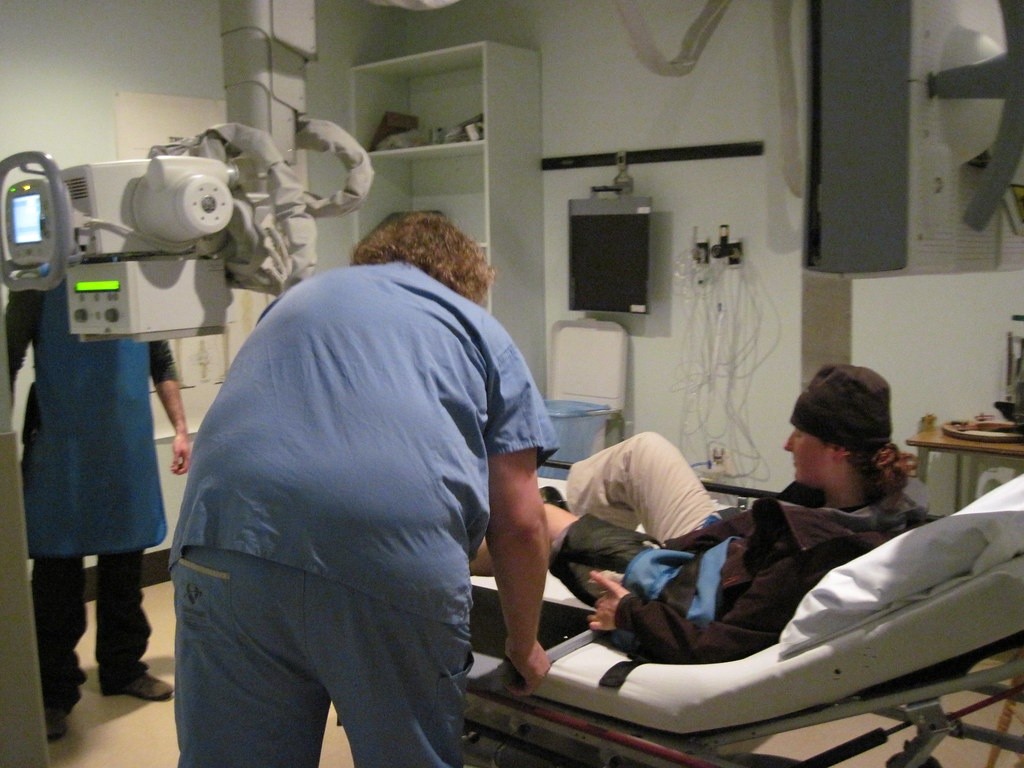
[779,477,989,661]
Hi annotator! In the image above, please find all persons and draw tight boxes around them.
[3,288,189,742]
[167,208,560,768]
[468,362,950,666]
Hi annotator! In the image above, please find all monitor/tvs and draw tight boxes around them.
[13,194,41,245]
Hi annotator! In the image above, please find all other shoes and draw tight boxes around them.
[45,712,67,740]
[122,673,173,699]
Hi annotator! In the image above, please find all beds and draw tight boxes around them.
[462,477,1024,768]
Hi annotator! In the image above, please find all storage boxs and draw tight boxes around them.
[537,318,628,480]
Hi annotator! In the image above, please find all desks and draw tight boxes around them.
[907,426,1024,482]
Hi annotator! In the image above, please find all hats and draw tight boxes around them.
[791,364,891,445]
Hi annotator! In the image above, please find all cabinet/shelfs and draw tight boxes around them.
[350,40,547,399]
